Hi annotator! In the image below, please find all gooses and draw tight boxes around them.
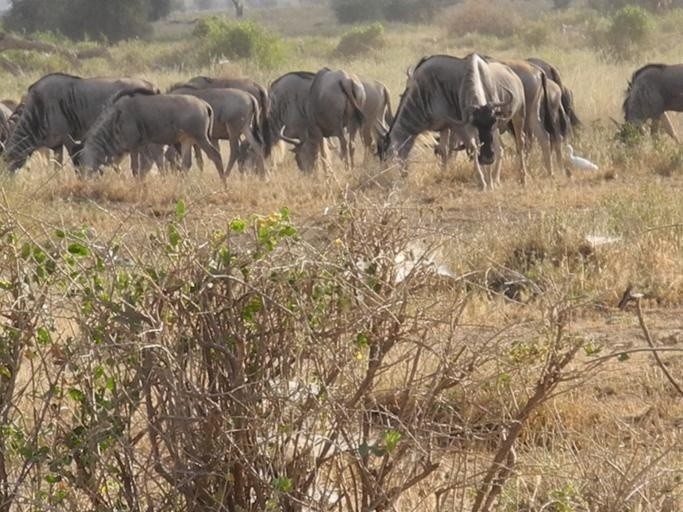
[564,144,599,171]
